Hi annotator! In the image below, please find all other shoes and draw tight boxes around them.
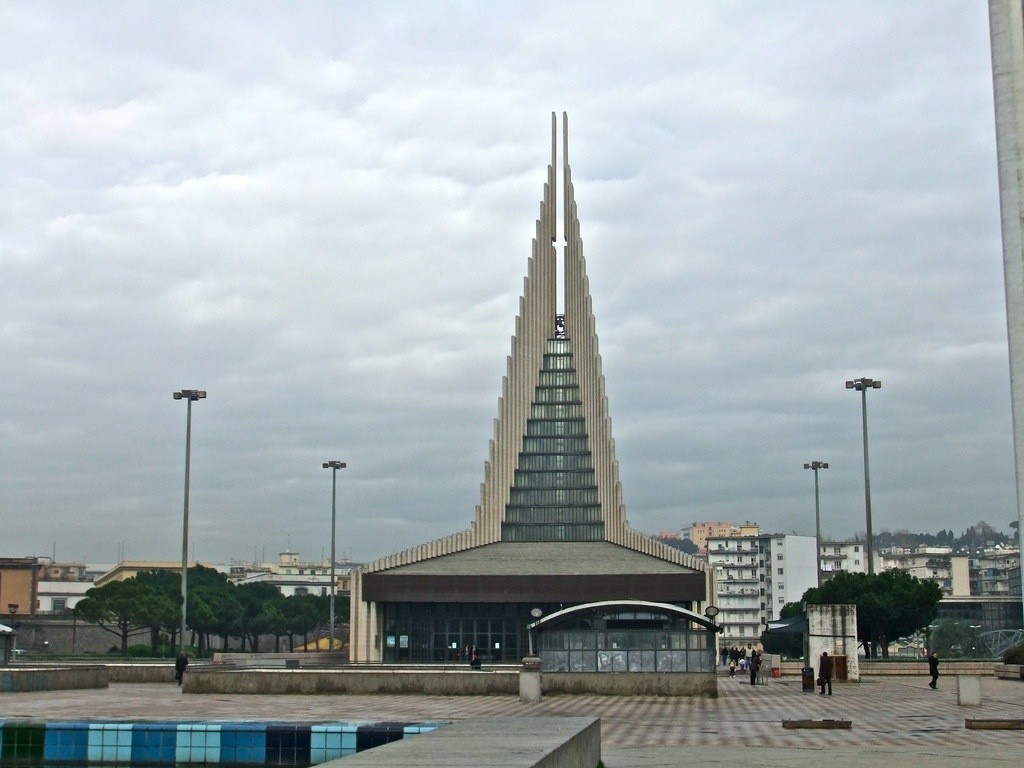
[928,683,938,689]
[829,692,831,695]
[820,692,825,694]
[733,675,736,678]
[730,676,732,678]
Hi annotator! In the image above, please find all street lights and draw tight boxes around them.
[323,460,348,651]
[173,389,207,654]
[804,461,829,588]
[847,377,881,572]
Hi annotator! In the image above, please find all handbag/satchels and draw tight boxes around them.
[739,659,746,665]
[175,672,179,680]
[817,676,825,686]
[734,668,736,672]
[749,664,759,671]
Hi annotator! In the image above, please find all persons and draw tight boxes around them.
[175,651,188,686]
[721,643,762,685]
[460,639,478,661]
[818,652,832,695]
[928,652,940,689]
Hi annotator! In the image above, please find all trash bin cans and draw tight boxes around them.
[771,667,779,678]
[801,667,815,692]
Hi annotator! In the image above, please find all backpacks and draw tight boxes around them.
[730,662,734,668]
